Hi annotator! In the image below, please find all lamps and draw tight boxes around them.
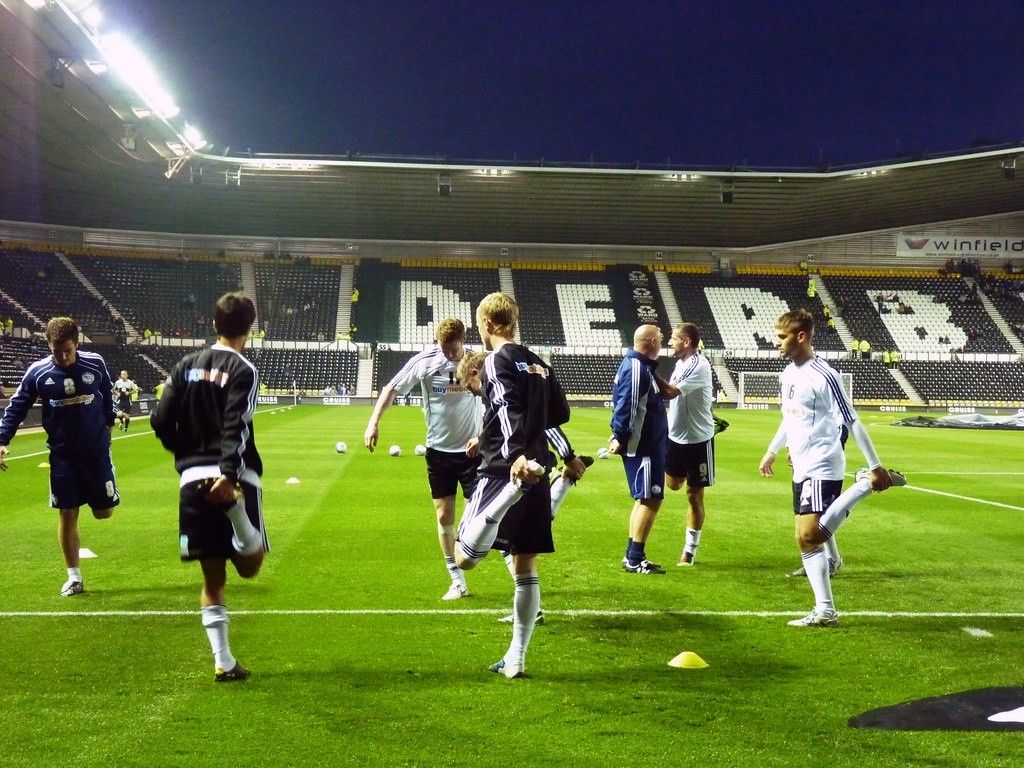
[119,123,137,151]
[226,165,242,190]
[164,159,187,181]
[44,48,80,89]
[720,180,735,204]
[1001,159,1016,179]
[437,172,452,198]
[190,164,205,185]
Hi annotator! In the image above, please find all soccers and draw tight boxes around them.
[415,445,425,455]
[597,447,609,459]
[389,445,401,456]
[336,441,347,453]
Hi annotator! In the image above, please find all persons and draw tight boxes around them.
[454,292,569,679]
[364,317,486,602]
[800,259,1024,368]
[248,330,256,338]
[608,324,668,575]
[258,328,265,339]
[0,317,121,597]
[653,324,729,567]
[279,287,359,341]
[299,389,306,395]
[150,294,270,682]
[454,352,593,625]
[786,423,848,578]
[758,310,908,626]
[259,382,266,394]
[324,382,356,396]
[0,316,167,432]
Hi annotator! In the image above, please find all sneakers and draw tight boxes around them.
[488,659,524,678]
[622,557,667,575]
[497,610,544,626]
[677,550,695,566]
[827,556,843,578]
[548,454,594,486]
[213,661,251,681]
[60,581,83,597]
[442,580,470,601]
[197,477,245,512]
[855,466,907,487]
[510,458,546,494]
[712,414,729,435]
[787,606,839,628]
[785,567,807,577]
